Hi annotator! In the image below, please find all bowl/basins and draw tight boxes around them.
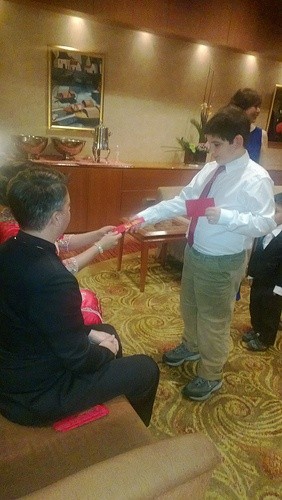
[51,137,86,155]
[14,133,48,155]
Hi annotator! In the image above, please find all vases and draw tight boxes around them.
[184,151,207,164]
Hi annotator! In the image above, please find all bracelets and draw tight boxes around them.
[95,243,104,254]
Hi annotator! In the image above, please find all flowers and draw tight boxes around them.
[176,102,213,154]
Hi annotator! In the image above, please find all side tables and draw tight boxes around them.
[117,216,187,293]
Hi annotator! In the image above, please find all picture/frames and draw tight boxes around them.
[47,45,107,132]
[266,84,282,149]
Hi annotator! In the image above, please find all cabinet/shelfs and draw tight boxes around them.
[15,154,282,234]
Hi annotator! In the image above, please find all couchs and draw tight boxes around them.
[0,395,226,500]
[156,186,282,264]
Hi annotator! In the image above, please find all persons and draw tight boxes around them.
[0,158,160,428]
[125,105,276,403]
[206,87,282,351]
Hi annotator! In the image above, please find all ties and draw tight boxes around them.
[187,166,225,248]
[263,234,271,249]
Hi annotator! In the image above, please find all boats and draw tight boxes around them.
[51,89,100,126]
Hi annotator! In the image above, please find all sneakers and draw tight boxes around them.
[247,338,270,350]
[242,331,260,342]
[184,376,224,400]
[163,343,200,366]
